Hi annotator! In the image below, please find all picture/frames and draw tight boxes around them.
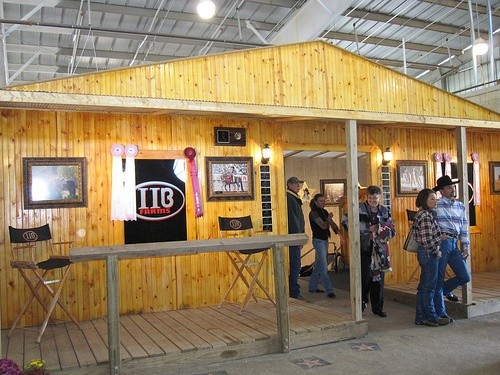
[206,155,253,201]
[318,178,347,206]
[397,160,428,197]
[488,161,500,195]
[21,157,87,207]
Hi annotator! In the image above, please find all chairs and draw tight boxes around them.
[8,222,81,342]
[218,212,275,310]
[407,208,449,283]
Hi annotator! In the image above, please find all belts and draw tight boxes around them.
[438,232,458,238]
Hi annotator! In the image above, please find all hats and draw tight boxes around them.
[303,187,309,194]
[288,177,304,183]
[433,175,460,192]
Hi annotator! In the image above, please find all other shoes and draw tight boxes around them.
[374,311,387,317]
[441,315,453,322]
[309,288,325,293]
[326,292,336,298]
[443,289,459,302]
[414,315,448,326]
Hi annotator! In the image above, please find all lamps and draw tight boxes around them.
[382,147,395,164]
[262,144,272,164]
[473,0,489,55]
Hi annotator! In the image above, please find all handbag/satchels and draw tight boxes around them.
[403,228,417,252]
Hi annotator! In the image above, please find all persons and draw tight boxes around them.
[308,194,347,298]
[341,186,395,316]
[412,188,453,326]
[432,176,470,321]
[287,177,306,300]
[228,162,235,183]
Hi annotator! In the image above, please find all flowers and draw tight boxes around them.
[0,358,50,375]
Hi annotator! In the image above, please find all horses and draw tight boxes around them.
[219,168,247,192]
